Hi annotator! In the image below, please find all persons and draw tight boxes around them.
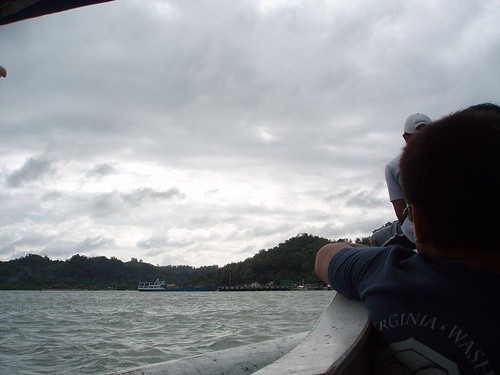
[313,102,499,374]
[369,113,433,247]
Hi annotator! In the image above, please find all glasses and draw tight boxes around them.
[401,203,413,223]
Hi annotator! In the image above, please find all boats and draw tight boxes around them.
[138,282,165,291]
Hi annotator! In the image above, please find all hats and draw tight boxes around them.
[404,112,433,134]
